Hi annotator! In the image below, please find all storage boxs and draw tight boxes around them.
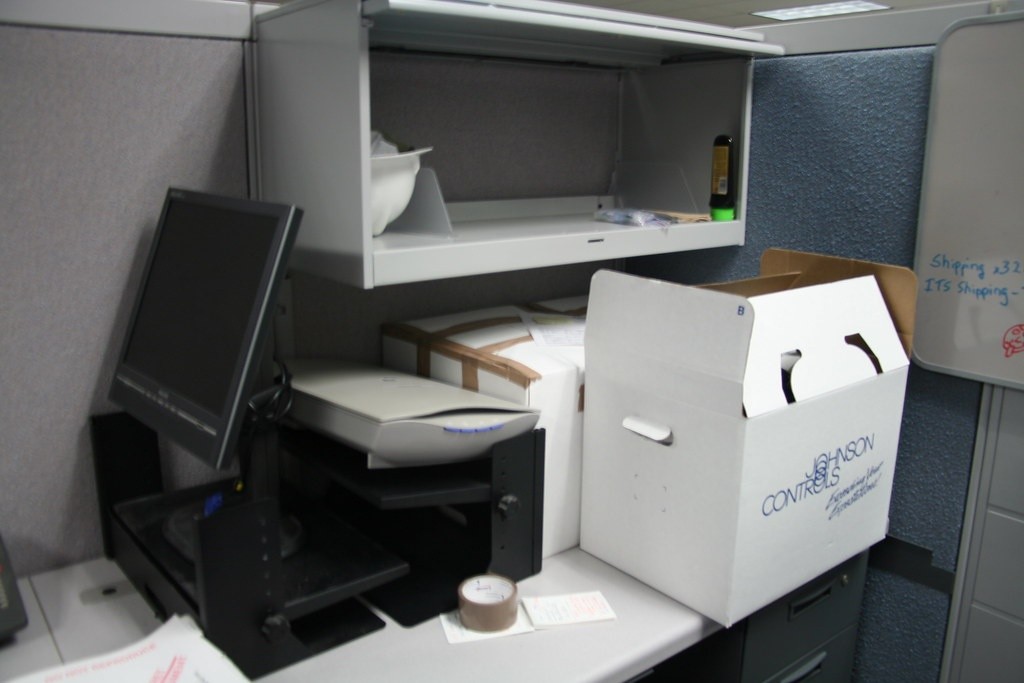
[370,291,589,559]
[569,245,920,629]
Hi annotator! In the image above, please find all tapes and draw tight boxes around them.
[456,572,519,634]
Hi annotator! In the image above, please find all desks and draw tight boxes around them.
[0,546,721,683]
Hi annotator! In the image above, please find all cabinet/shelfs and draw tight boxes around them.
[255,0,786,295]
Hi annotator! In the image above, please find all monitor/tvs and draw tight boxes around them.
[108,186,302,567]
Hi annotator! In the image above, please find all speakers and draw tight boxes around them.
[197,502,301,681]
[91,410,163,560]
